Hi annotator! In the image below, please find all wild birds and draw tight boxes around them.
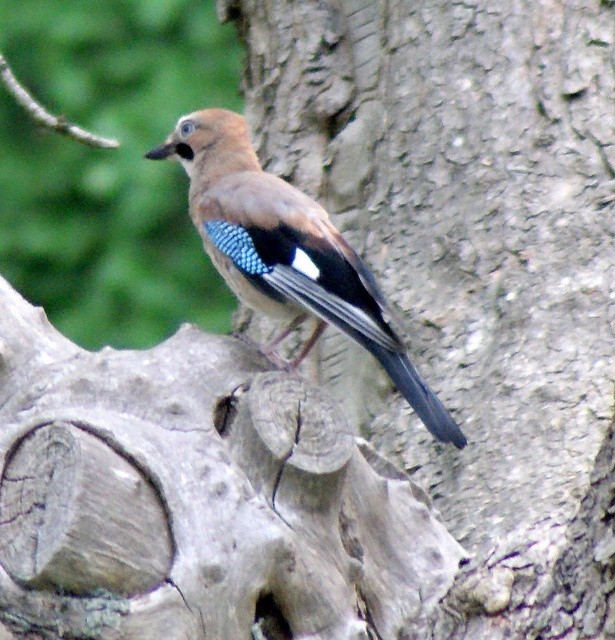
[143,108,467,450]
